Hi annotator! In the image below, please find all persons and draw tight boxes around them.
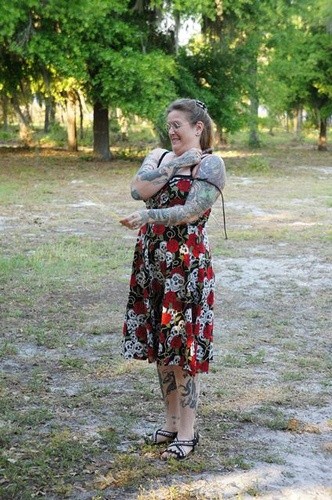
[119,97,228,463]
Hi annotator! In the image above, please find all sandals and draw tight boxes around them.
[139,427,178,446]
[159,431,200,461]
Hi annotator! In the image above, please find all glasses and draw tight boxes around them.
[166,120,195,131]
